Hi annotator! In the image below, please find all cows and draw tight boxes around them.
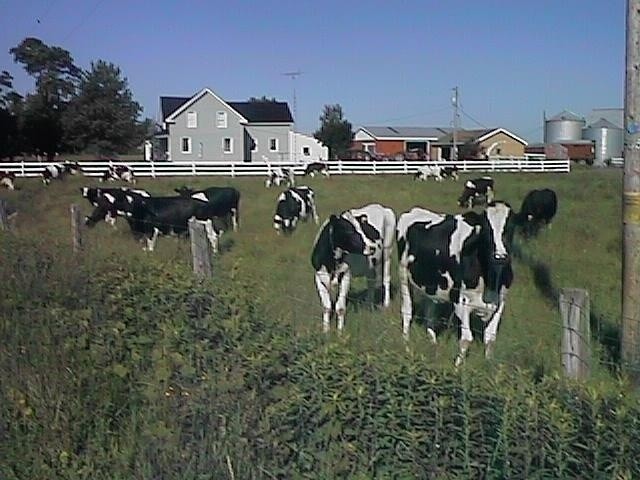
[455,176,497,207]
[311,201,397,334]
[272,185,320,235]
[99,165,136,185]
[40,161,78,185]
[412,165,446,185]
[515,190,558,241]
[264,167,296,188]
[395,199,533,367]
[80,184,128,208]
[116,191,219,251]
[434,165,460,182]
[83,188,152,228]
[170,185,241,232]
[64,159,80,176]
[0,173,14,190]
[304,162,328,180]
